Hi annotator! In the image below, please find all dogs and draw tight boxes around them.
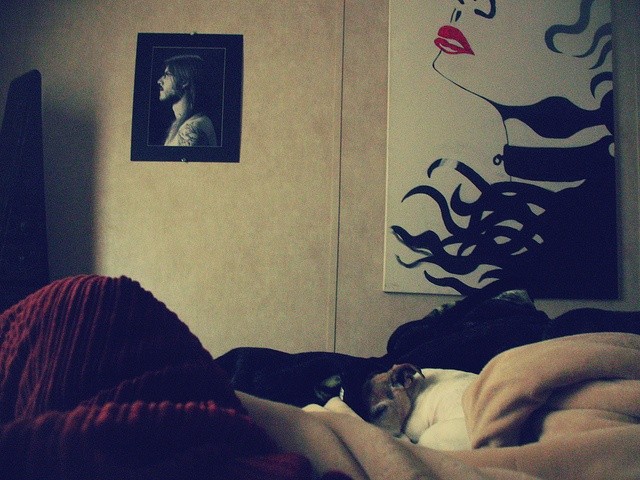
[357,361,520,453]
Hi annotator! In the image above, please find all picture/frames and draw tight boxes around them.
[130,33,244,163]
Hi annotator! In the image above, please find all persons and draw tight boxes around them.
[154,53,216,149]
